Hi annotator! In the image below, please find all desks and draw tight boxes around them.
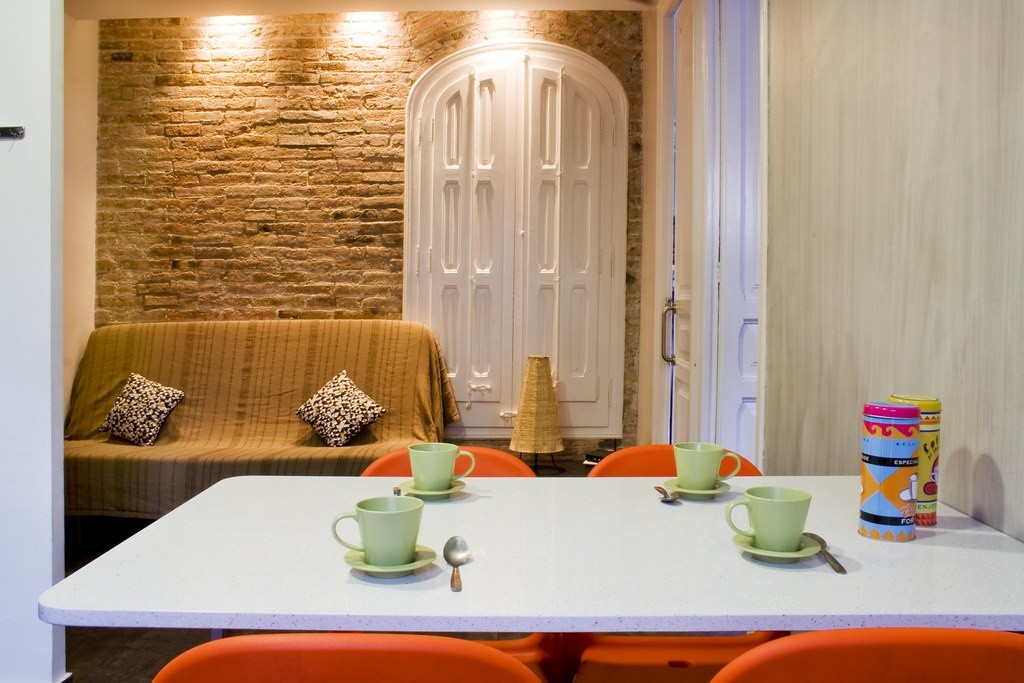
[35,475,1024,634]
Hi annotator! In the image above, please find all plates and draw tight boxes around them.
[398,478,466,500]
[732,531,822,564]
[664,477,732,500]
[344,543,436,577]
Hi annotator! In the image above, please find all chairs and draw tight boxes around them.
[153,445,1024,683]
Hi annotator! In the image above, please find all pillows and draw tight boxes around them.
[294,369,386,448]
[99,372,185,446]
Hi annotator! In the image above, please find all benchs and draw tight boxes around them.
[64,319,460,520]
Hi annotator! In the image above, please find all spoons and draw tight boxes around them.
[654,485,680,503]
[443,536,470,592]
[802,532,847,575]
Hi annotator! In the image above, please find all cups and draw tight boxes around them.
[725,485,812,551]
[331,496,425,565]
[408,442,476,490]
[673,442,741,489]
